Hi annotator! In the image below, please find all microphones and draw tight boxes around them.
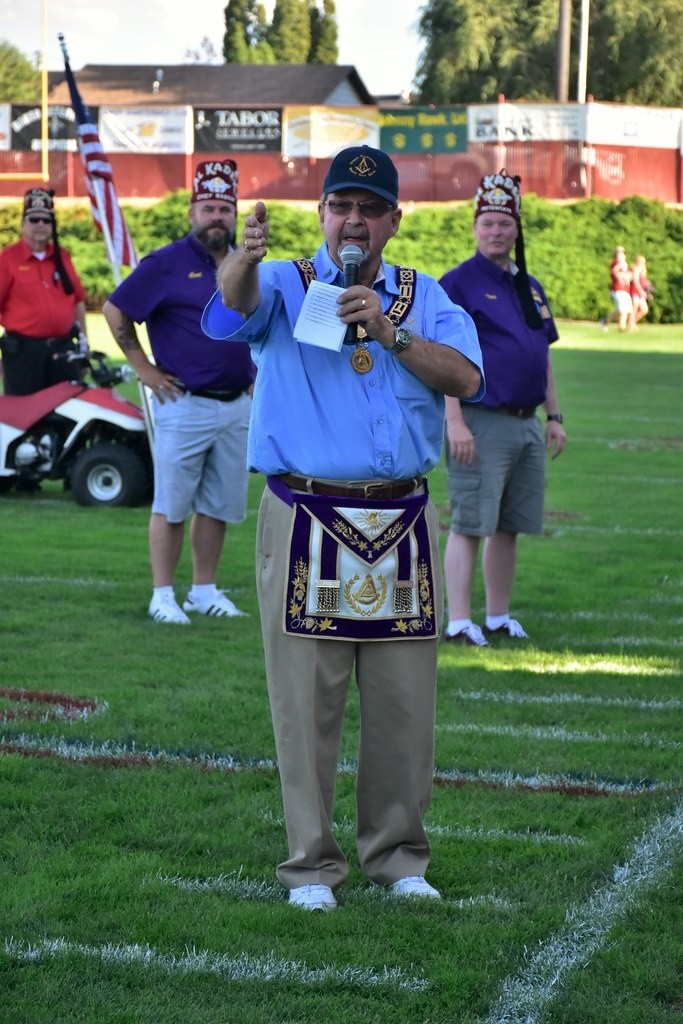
[339,245,364,347]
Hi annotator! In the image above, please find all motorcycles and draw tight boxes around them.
[0,320,155,506]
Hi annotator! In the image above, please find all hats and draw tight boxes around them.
[191,159,238,208]
[23,188,73,295]
[323,145,398,208]
[475,169,543,329]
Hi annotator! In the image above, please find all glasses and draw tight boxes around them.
[319,199,394,218]
[25,215,53,224]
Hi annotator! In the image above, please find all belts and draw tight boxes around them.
[460,400,536,419]
[6,329,72,348]
[276,473,422,499]
[169,380,242,402]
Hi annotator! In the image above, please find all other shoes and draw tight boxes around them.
[148,593,191,625]
[288,884,337,913]
[444,624,489,647]
[391,876,441,900]
[183,589,251,618]
[482,619,530,640]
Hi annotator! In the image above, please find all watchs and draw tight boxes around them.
[385,326,412,355]
[547,413,562,424]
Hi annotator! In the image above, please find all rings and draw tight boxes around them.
[245,239,249,247]
[159,385,164,389]
[362,300,366,307]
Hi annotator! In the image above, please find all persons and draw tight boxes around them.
[201,144,487,914]
[0,186,89,492]
[436,168,567,647]
[601,245,651,333]
[102,158,258,625]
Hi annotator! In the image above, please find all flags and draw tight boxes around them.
[65,63,140,269]
